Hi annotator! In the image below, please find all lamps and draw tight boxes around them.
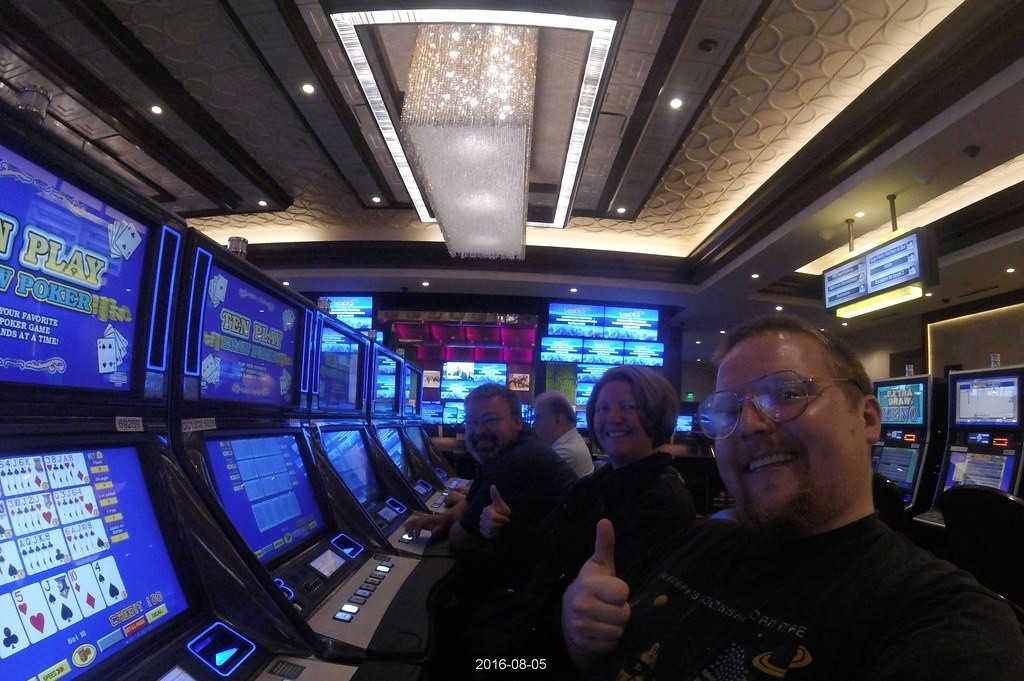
[400,22,540,261]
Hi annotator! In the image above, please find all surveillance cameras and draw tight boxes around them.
[964,145,980,158]
[700,40,718,52]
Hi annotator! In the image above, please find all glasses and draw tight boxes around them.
[465,413,511,426]
[698,370,865,441]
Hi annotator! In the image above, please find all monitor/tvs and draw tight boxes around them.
[942,375,1022,492]
[871,382,926,492]
[676,415,694,432]
[540,299,665,428]
[0,121,537,681]
[822,227,941,314]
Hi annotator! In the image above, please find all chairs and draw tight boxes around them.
[872,471,904,538]
[940,485,1024,610]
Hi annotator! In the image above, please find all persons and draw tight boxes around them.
[403,383,580,550]
[532,391,594,478]
[561,314,1024,681]
[478,366,697,681]
